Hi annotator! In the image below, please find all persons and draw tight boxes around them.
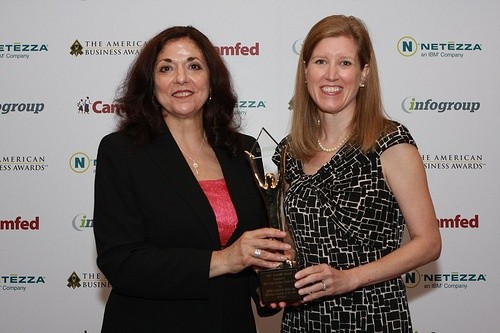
[251,14,442,333]
[93,26,318,333]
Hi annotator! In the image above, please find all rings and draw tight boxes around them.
[254,248,262,259]
[322,280,327,290]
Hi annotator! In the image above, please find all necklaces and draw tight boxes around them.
[317,119,351,152]
[169,128,206,175]
